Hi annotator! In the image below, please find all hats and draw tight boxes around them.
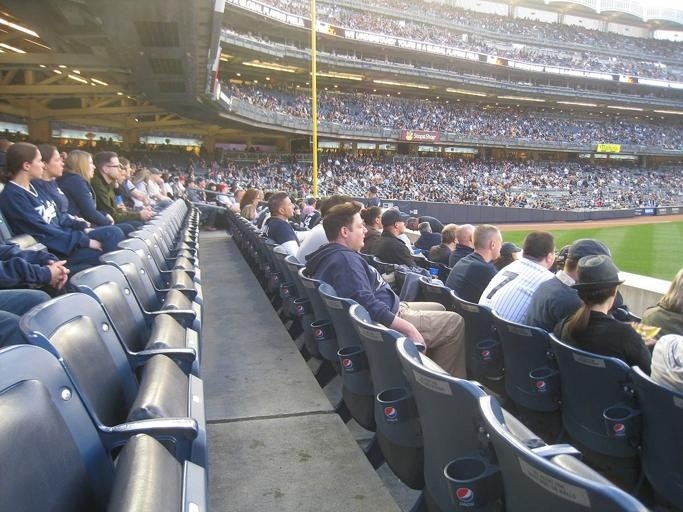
[568,254,627,294]
[499,242,521,255]
[380,206,410,226]
[567,238,612,261]
[555,245,572,262]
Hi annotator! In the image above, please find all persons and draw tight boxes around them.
[0,1,683,392]
